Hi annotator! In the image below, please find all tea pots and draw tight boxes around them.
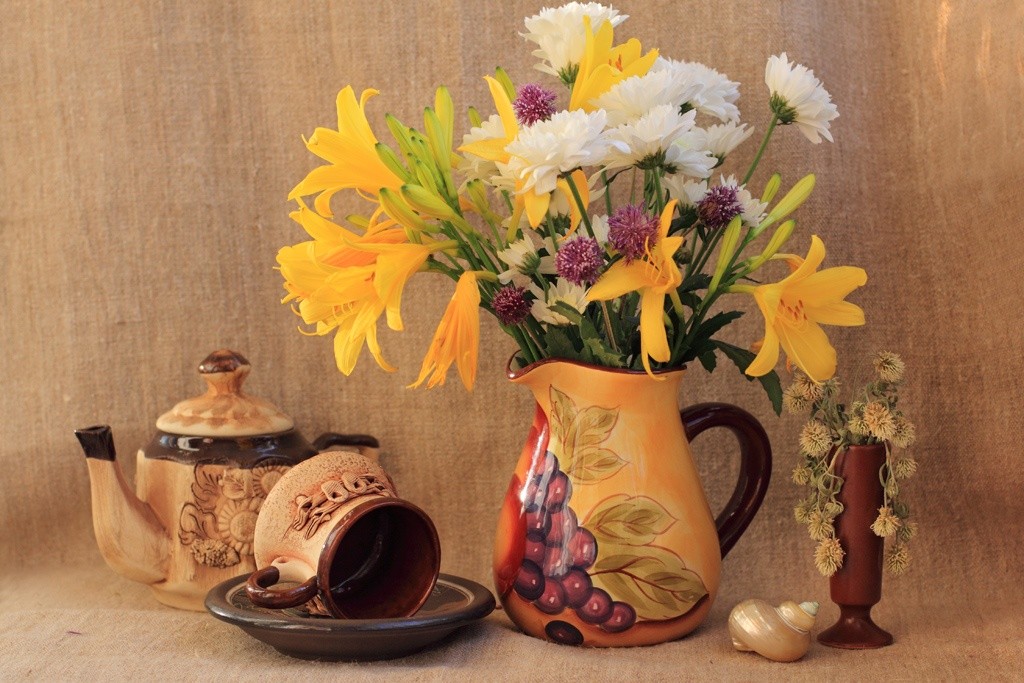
[74,349,380,612]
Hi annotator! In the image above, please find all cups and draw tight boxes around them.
[245,451,442,618]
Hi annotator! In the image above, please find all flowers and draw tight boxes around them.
[273,1,867,392]
[782,350,918,578]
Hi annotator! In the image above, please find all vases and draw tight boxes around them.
[492,350,772,649]
[817,438,895,650]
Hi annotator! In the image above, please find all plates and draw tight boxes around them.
[204,574,498,662]
